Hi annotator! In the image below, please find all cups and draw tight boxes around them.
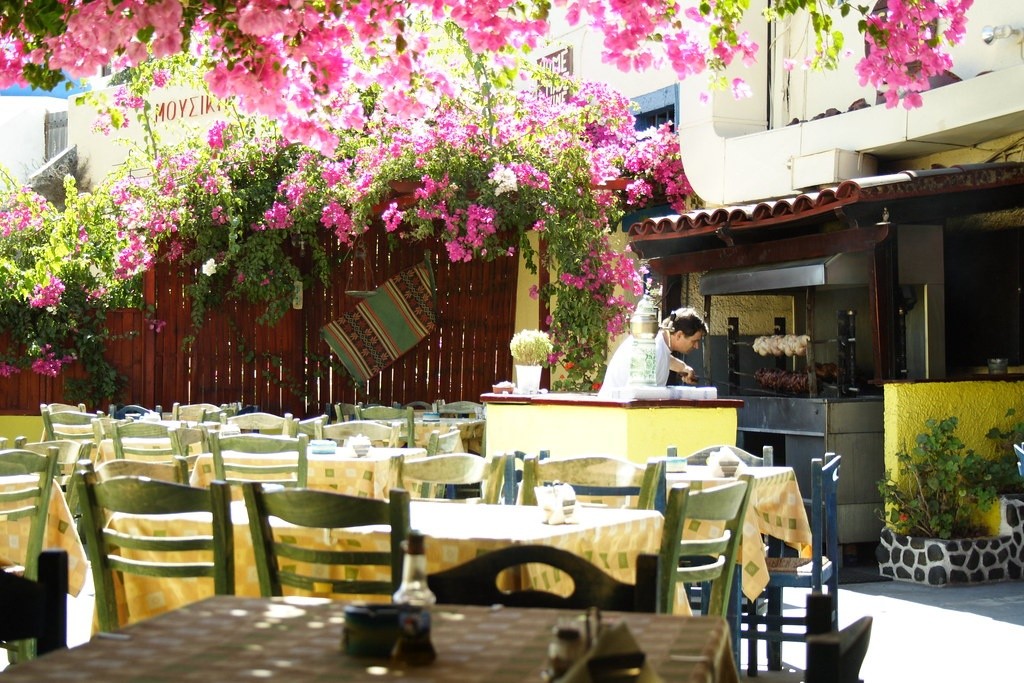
[987,358,1008,375]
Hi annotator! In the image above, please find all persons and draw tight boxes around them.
[598,307,709,397]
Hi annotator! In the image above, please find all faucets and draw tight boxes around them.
[658,310,677,333]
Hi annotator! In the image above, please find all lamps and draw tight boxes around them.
[981,24,1024,46]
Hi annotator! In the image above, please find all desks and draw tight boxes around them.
[369,415,484,446]
[0,474,88,598]
[0,595,741,683]
[38,424,96,443]
[191,446,429,500]
[668,465,814,674]
[89,501,694,640]
[93,437,202,481]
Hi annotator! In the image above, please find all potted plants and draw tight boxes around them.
[510,328,552,392]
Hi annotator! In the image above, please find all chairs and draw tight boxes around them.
[0,401,845,678]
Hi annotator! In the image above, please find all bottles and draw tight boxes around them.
[391,534,436,667]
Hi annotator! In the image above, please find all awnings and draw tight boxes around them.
[629,161,1024,273]
